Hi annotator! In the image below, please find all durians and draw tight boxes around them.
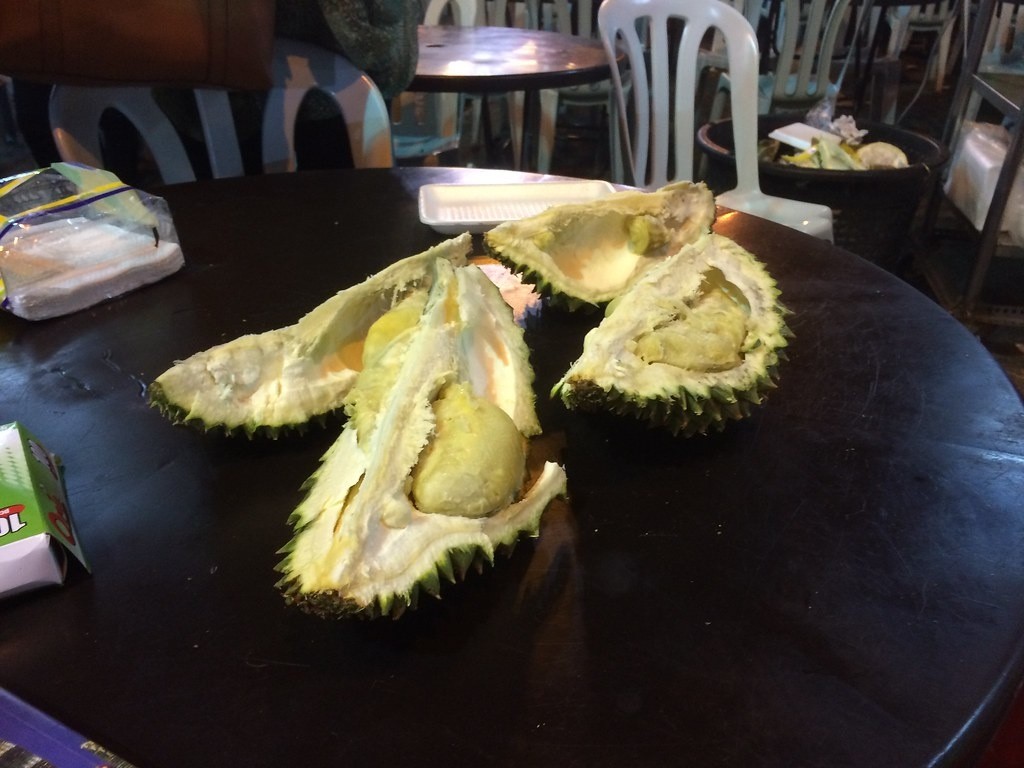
[551,234,793,438]
[145,241,543,442]
[485,181,720,312]
[279,259,570,617]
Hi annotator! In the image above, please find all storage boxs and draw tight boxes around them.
[0,420,91,599]
[0,687,139,768]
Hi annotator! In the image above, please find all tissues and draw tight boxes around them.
[0,162,186,321]
[946,121,1022,229]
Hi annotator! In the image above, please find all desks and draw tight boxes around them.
[0,163,1024,768]
[381,25,627,174]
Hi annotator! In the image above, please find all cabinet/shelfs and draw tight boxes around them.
[897,0,1023,339]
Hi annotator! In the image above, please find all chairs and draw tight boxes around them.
[48,0,1024,243]
